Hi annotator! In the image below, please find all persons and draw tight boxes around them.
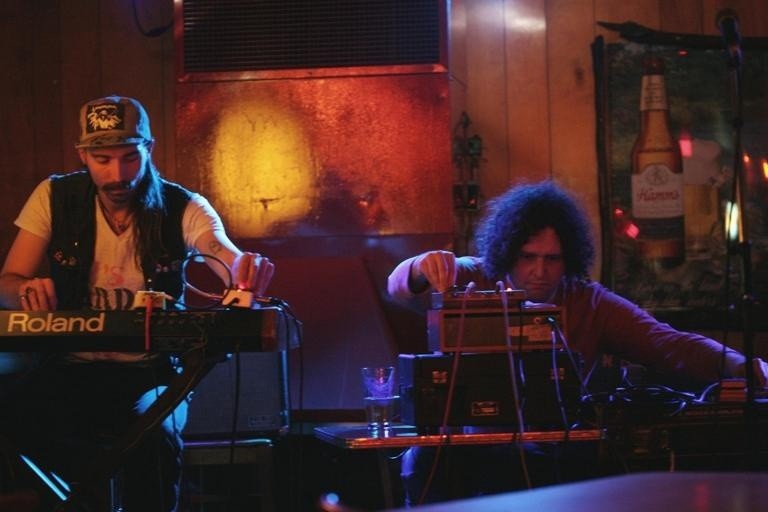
[0,91,276,511]
[381,173,768,400]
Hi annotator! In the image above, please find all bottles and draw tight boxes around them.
[628,59,686,285]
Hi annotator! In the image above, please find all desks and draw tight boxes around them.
[314,423,604,510]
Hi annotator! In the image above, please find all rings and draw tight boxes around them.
[24,287,35,295]
[18,293,27,302]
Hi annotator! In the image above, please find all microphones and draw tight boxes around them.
[715,6,743,65]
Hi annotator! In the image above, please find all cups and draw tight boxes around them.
[360,366,400,430]
[683,140,726,260]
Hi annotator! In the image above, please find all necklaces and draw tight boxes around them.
[99,202,135,235]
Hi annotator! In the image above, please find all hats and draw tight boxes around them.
[74,97,152,149]
